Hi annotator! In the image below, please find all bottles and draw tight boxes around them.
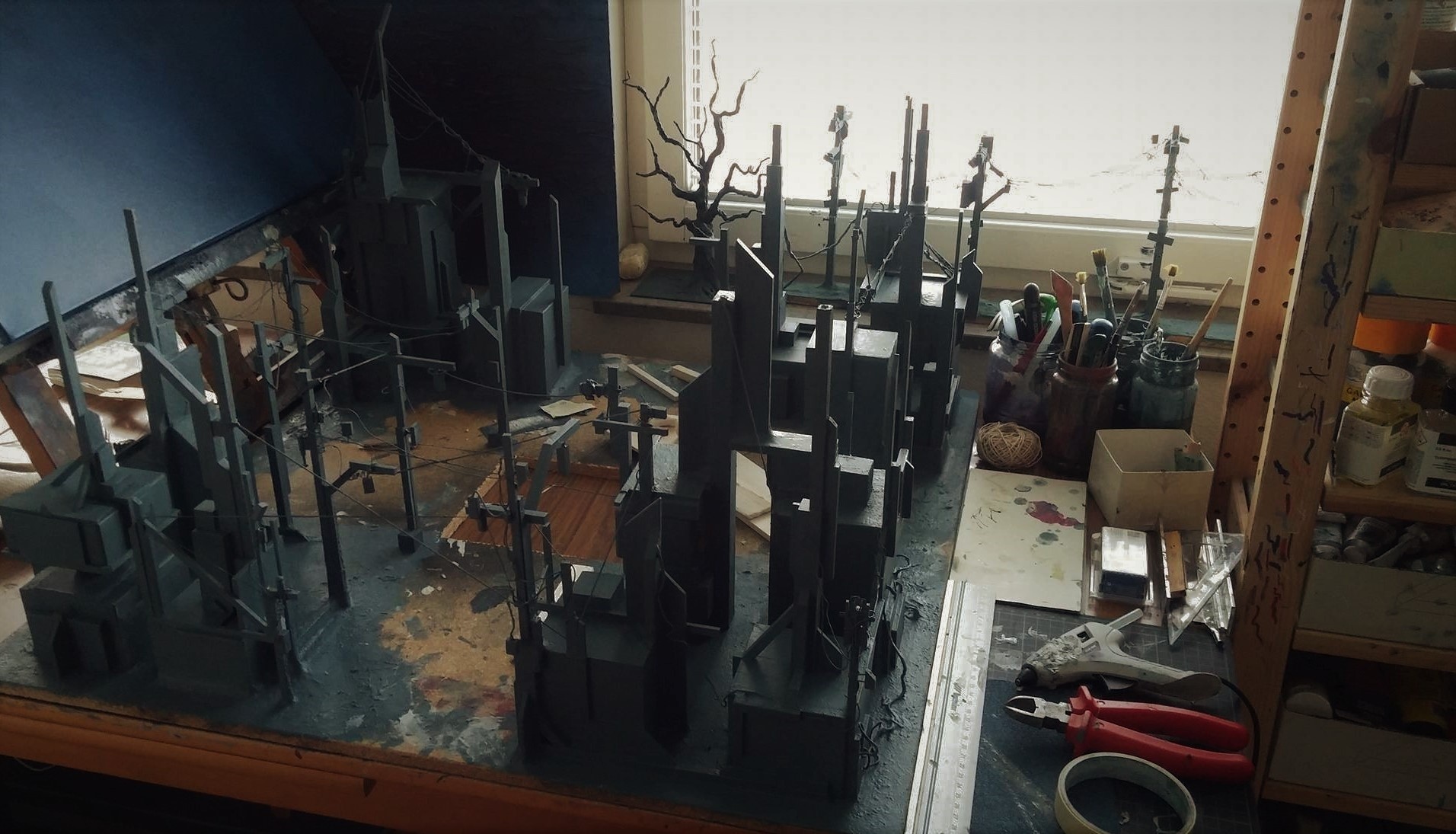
[984,328,1065,433]
[1114,316,1165,430]
[1404,376,1456,501]
[1335,316,1422,429]
[1035,350,1119,479]
[1334,365,1423,489]
[1126,341,1200,435]
[1412,322,1456,414]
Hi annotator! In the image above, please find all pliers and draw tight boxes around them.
[1005,686,1252,779]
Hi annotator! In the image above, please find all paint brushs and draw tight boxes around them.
[1180,277,1234,354]
[1147,264,1179,335]
[1075,272,1088,323]
[1091,249,1120,330]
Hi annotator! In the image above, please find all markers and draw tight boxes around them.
[1024,282,1045,343]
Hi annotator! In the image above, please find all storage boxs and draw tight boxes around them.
[1266,702,1456,813]
[1293,555,1456,652]
[1395,63,1456,169]
[1088,428,1214,531]
[1363,219,1456,301]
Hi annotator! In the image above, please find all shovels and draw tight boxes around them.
[1049,269,1072,331]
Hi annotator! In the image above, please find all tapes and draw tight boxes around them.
[1054,753,1197,834]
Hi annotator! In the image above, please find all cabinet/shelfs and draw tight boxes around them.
[1206,0,1456,834]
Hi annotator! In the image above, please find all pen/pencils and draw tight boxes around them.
[1064,279,1147,369]
